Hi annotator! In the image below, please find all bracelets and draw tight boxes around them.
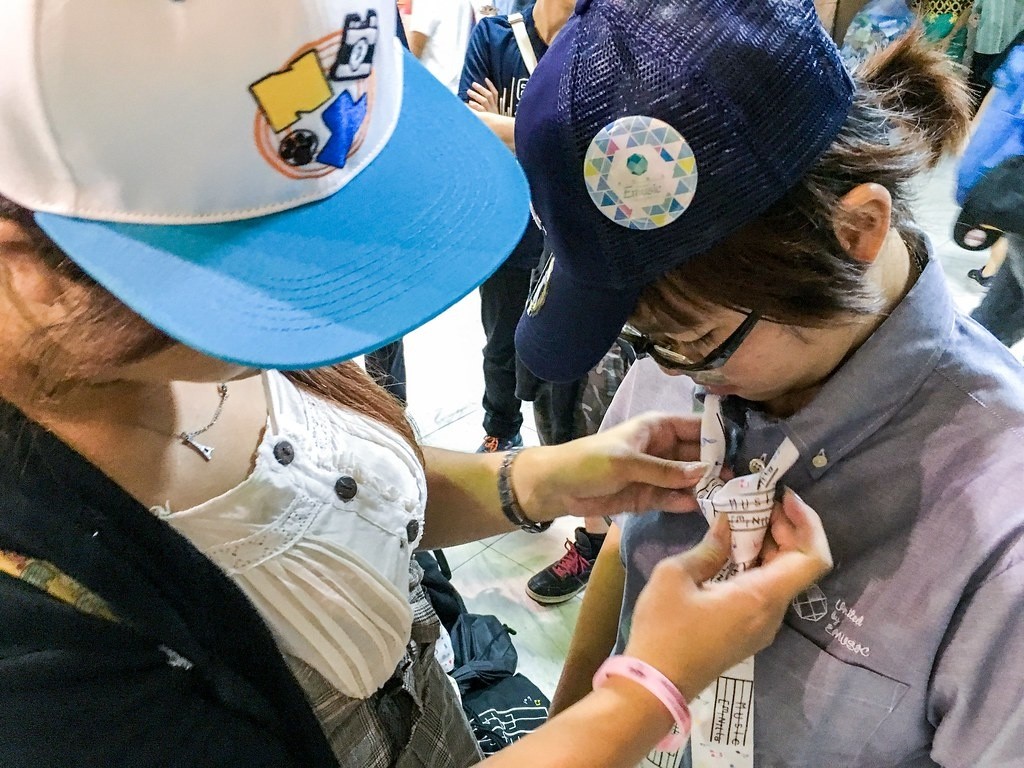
[592,655,692,752]
[499,447,554,534]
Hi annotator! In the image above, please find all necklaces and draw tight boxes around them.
[135,383,230,461]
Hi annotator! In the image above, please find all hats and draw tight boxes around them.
[513,0,861,381]
[0,0,532,373]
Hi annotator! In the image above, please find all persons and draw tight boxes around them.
[363,0,1023,611]
[516,0,1024,767]
[0,0,837,768]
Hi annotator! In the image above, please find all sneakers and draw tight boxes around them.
[525,527,596,604]
[475,431,523,453]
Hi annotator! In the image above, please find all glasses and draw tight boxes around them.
[615,309,762,371]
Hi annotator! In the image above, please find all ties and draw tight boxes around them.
[630,392,800,768]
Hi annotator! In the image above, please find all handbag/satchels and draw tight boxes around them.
[449,612,552,755]
